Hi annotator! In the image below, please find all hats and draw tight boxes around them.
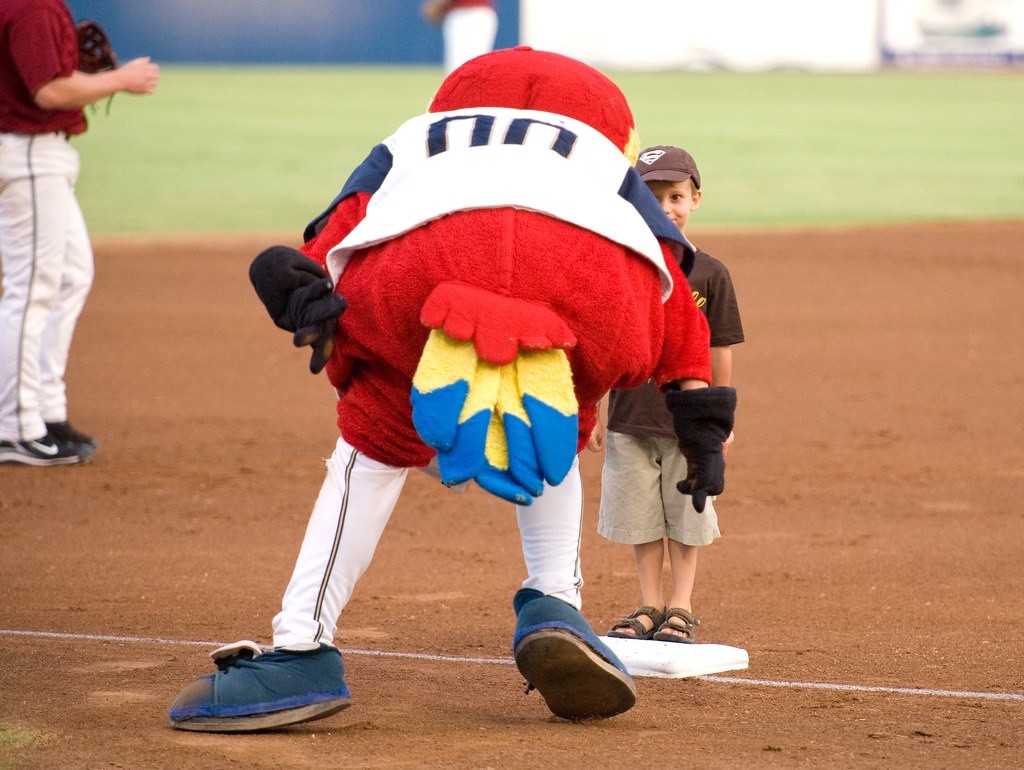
[634,143,702,192]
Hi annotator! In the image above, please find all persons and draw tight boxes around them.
[587,146,745,643]
[168,45,738,733]
[0,0,159,467]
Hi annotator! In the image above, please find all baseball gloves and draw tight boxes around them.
[76,20,115,75]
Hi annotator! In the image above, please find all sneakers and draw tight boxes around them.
[0,434,94,469]
[45,418,96,444]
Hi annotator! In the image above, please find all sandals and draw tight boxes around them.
[606,605,668,639]
[653,607,702,645]
